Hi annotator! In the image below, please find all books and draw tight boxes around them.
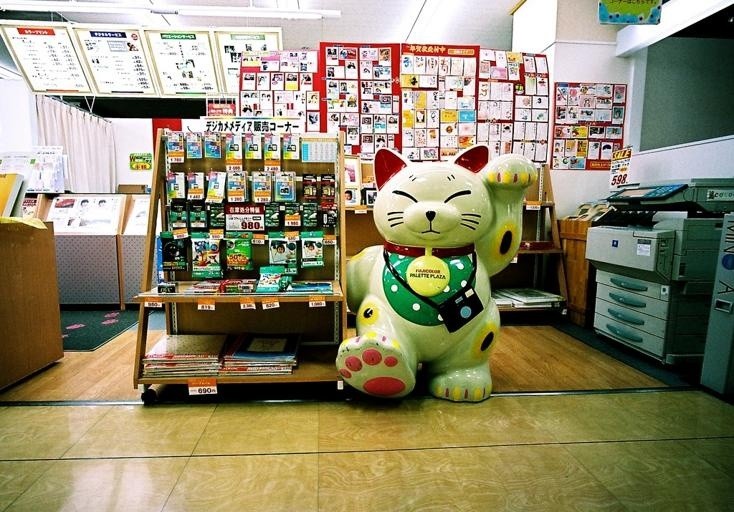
[140,334,301,377]
[491,288,566,312]
[0,174,22,217]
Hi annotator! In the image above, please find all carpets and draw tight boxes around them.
[58,308,155,353]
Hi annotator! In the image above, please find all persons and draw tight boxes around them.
[79,199,88,226]
[344,190,356,205]
[271,239,323,264]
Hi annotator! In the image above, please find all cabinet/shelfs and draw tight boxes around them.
[1,217,64,392]
[131,128,348,404]
[343,162,571,316]
